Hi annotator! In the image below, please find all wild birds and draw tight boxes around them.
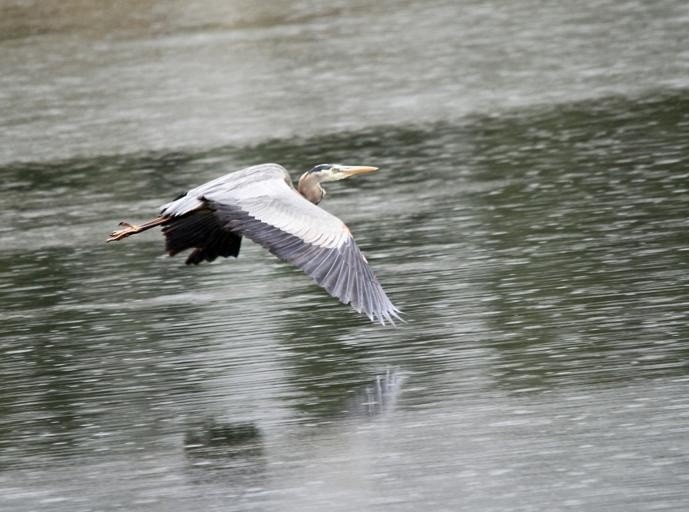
[106,162,410,329]
